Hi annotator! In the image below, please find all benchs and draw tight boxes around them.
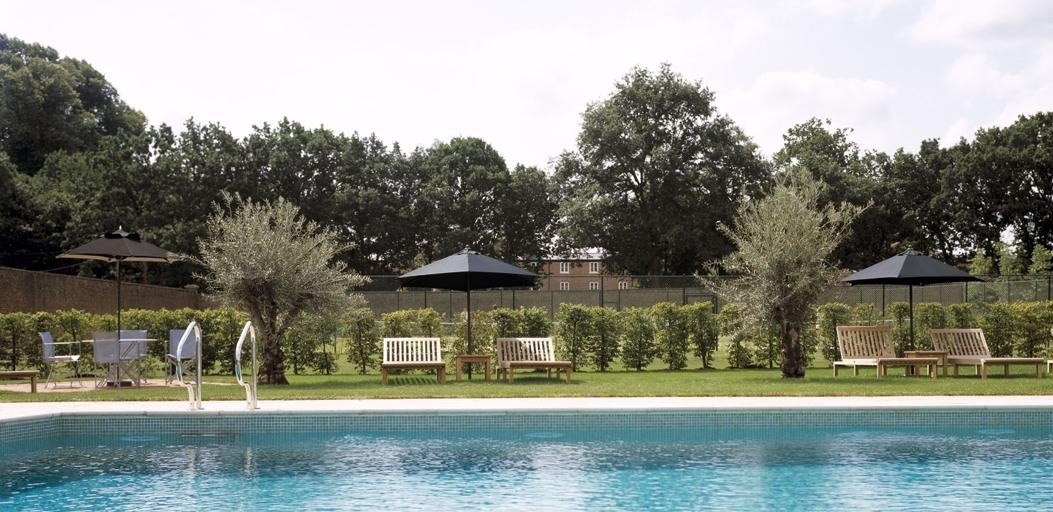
[930,328,1045,378]
[833,325,938,380]
[0,370,41,394]
[495,336,571,383]
[382,338,446,384]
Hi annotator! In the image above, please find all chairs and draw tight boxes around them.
[38,328,200,390]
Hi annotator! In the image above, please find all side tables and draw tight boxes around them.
[904,349,950,379]
[455,355,492,381]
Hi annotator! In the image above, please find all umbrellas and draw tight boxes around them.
[837,241,986,373]
[394,242,542,380]
[52,224,187,380]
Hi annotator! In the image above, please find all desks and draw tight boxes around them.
[1047,360,1053,373]
